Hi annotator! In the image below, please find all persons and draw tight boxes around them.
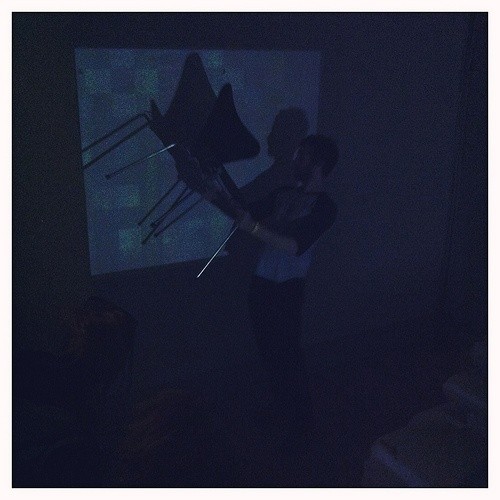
[13,295,487,489]
[228,133,342,463]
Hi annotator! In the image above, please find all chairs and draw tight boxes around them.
[101,81,262,282]
